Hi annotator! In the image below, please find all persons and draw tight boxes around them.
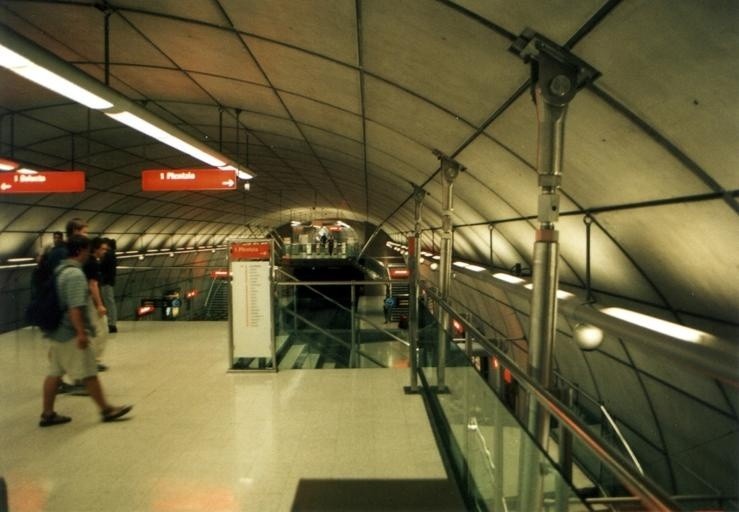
[385,294,395,322]
[383,300,388,323]
[327,236,334,257]
[320,233,326,249]
[33,219,134,428]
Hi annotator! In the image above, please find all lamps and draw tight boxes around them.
[0,1,257,182]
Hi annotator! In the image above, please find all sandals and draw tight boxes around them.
[37,409,73,427]
[98,404,136,423]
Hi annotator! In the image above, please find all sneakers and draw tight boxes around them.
[76,383,89,396]
[56,381,75,395]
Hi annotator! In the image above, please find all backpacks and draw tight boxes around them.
[25,265,79,331]
